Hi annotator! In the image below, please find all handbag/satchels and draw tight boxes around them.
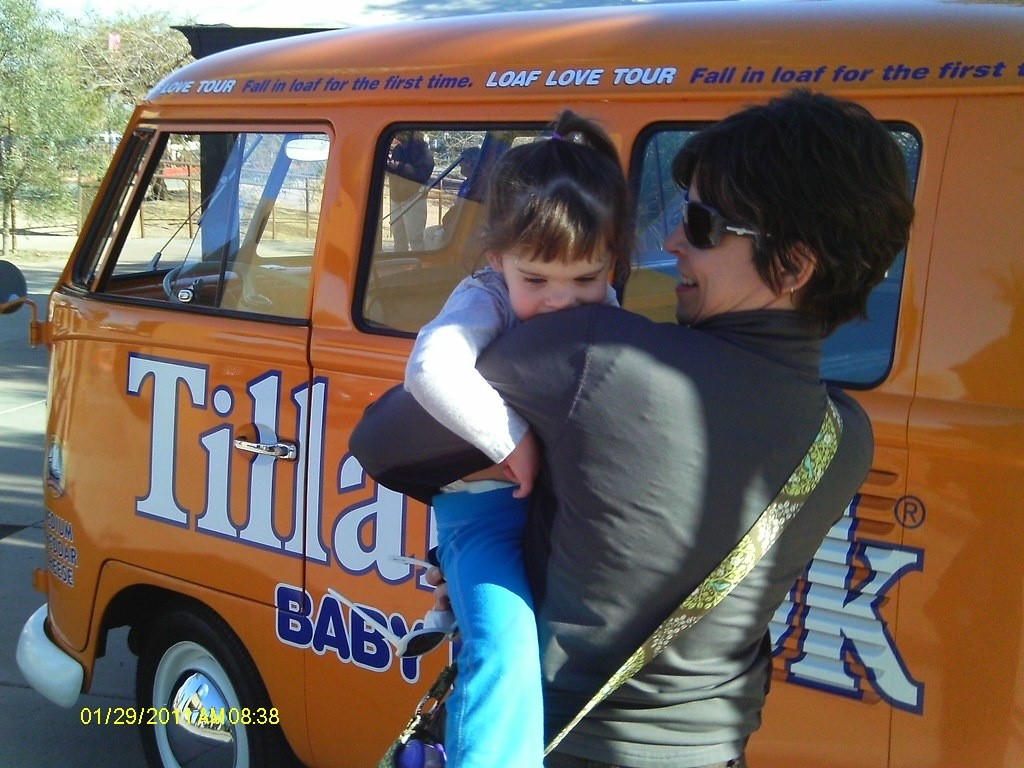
[379,713,442,768]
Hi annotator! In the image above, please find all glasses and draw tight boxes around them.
[680,194,774,250]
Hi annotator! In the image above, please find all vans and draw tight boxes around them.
[0,0,1024,768]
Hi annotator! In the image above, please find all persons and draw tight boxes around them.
[441,146,478,247]
[404,110,642,768]
[346,87,914,768]
[390,129,434,254]
